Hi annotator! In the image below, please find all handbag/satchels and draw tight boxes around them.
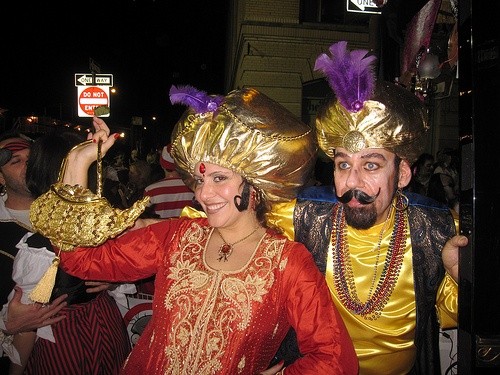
[28,138,150,247]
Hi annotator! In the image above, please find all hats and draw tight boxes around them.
[169,84,315,202]
[313,42,428,167]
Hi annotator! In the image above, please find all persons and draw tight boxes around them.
[116,38,468,375]
[0,134,162,375]
[50,86,360,375]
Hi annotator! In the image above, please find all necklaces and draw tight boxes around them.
[215,225,261,263]
[333,200,407,320]
